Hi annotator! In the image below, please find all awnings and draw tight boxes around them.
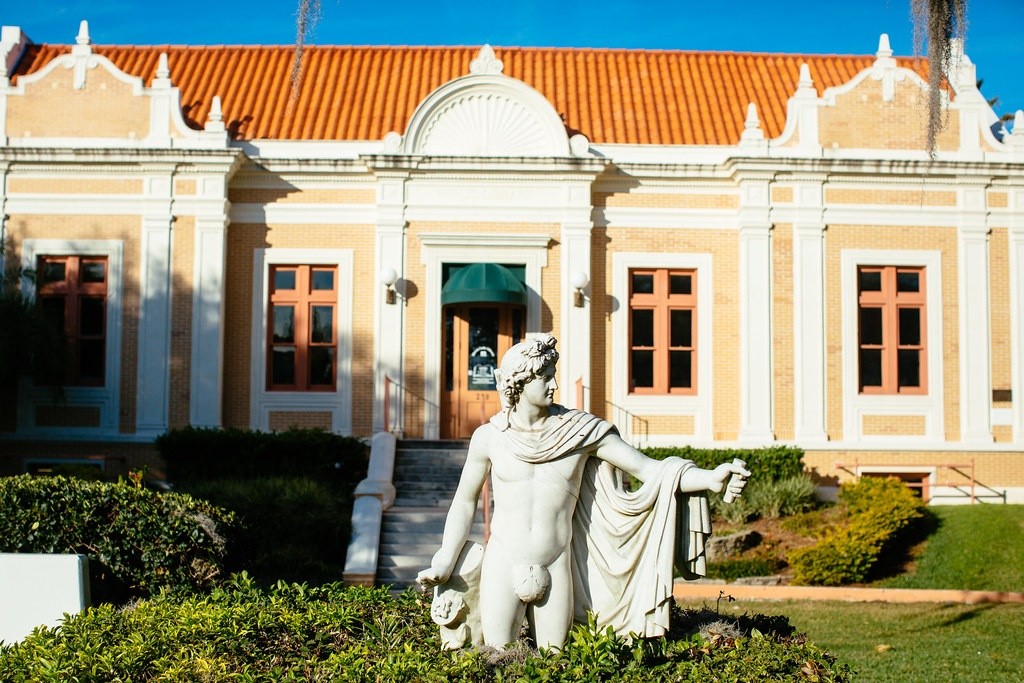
[441,262,527,311]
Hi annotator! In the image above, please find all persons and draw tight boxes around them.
[415,335,751,657]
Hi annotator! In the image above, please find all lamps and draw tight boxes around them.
[572,271,588,307]
[381,268,398,304]
